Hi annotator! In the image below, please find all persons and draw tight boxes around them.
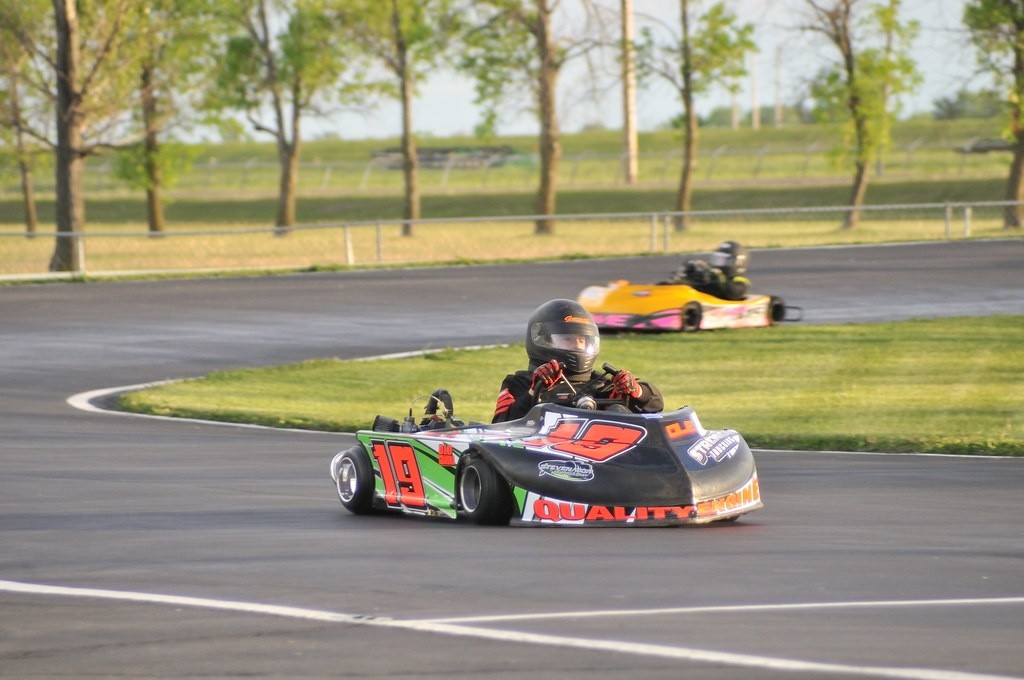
[683,240,750,300]
[491,298,664,426]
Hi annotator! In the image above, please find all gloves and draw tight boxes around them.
[688,260,709,271]
[611,369,640,398]
[531,359,562,388]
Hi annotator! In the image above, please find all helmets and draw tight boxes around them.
[709,241,750,277]
[526,299,600,381]
